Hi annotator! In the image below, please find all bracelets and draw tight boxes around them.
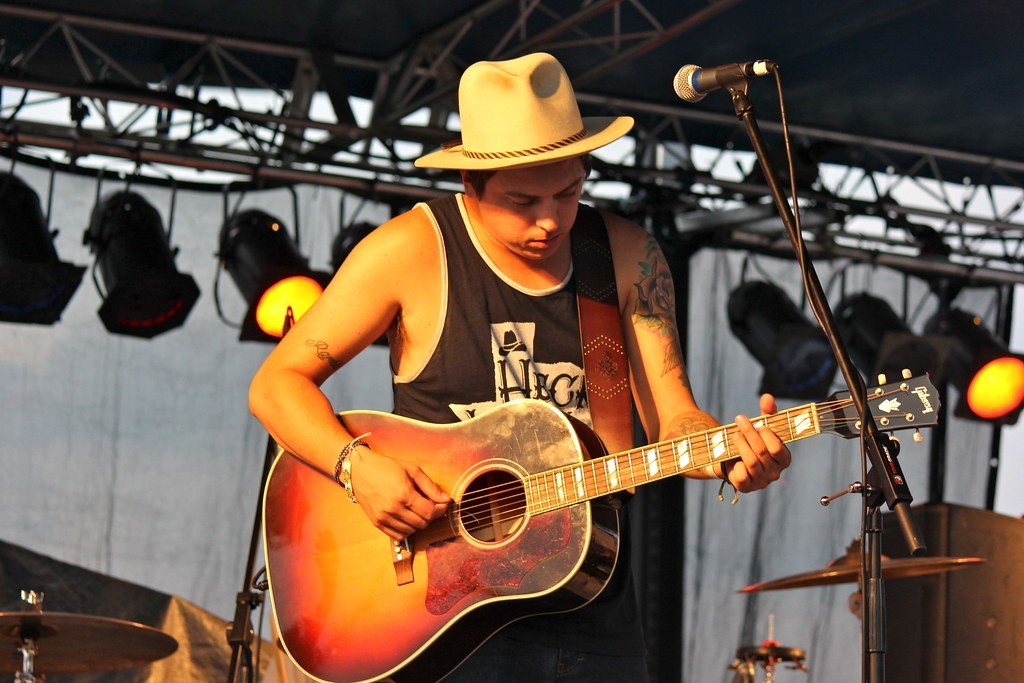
[707,459,739,506]
[335,432,371,503]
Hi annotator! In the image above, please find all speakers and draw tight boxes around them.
[881,501,1024,683]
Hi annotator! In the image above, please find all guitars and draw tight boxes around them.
[262,367,942,683]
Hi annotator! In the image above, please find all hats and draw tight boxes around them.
[413,53,634,171]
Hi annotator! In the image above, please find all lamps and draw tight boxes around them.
[726,274,1023,423]
[0,171,390,343]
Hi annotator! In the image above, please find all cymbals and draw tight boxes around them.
[0,610,181,671]
[736,550,987,593]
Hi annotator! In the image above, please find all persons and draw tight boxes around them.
[248,52,791,683]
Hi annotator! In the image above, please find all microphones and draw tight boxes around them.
[672,58,776,103]
